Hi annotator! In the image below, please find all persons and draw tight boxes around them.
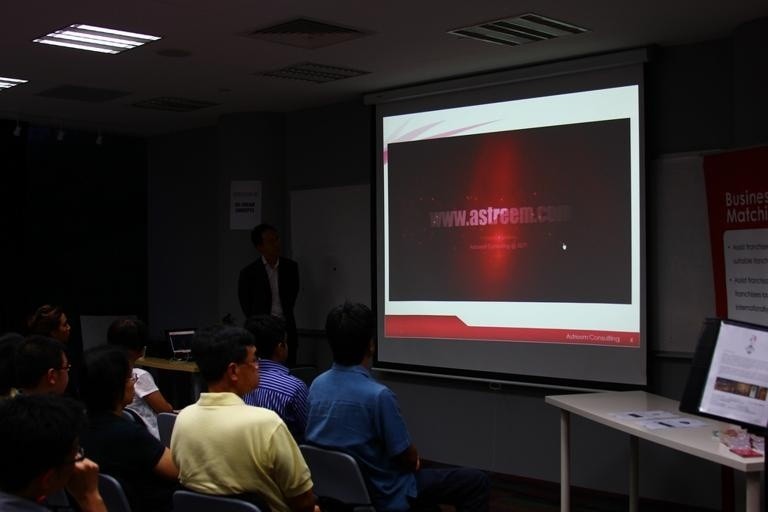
[238,223,300,368]
[0,302,493,512]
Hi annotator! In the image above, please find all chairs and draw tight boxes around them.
[156,411,178,449]
[123,409,135,422]
[299,444,377,512]
[172,489,261,512]
[64,473,132,512]
[1,493,50,512]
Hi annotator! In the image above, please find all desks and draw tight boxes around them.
[134,356,200,401]
[544,390,767,512]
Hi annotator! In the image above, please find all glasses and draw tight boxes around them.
[59,447,86,465]
[56,364,71,372]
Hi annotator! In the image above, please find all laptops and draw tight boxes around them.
[167,328,196,359]
